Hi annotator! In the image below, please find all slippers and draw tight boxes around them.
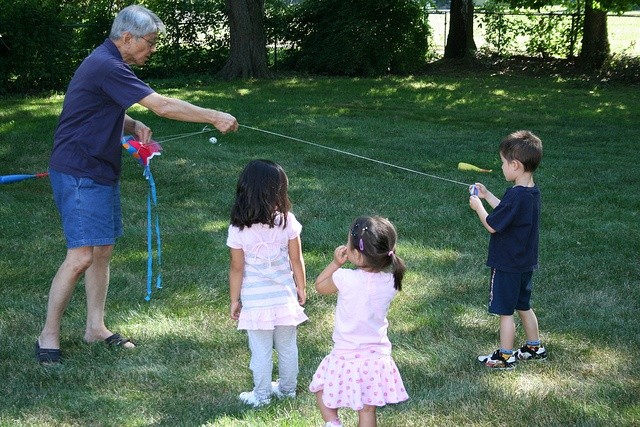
[82,332,136,353]
[35,338,64,369]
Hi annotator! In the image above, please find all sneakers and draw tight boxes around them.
[239,389,271,408]
[512,343,547,361]
[477,349,517,372]
[271,379,297,400]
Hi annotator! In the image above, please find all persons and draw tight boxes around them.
[36,7,239,370]
[308,216,409,427]
[469,130,547,369]
[226,158,307,406]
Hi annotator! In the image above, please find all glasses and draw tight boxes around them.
[140,35,161,48]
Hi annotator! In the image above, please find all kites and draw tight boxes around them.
[1,134,163,301]
